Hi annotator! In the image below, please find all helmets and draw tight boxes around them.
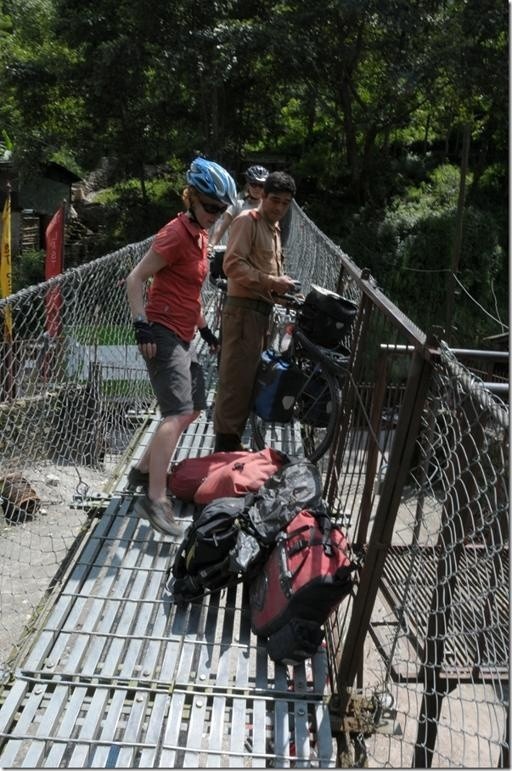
[245,165,269,184]
[186,157,238,210]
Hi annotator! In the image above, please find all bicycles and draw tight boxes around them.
[247,288,350,464]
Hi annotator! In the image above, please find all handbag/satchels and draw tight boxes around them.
[297,290,358,349]
[167,449,324,603]
[251,345,301,423]
[295,359,332,428]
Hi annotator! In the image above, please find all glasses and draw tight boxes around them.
[250,183,264,189]
[199,201,227,214]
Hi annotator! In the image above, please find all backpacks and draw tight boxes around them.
[246,508,357,665]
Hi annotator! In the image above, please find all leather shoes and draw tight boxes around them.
[134,493,182,537]
[127,466,171,487]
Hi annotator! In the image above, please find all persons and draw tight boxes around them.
[125,156,238,539]
[209,166,271,370]
[211,172,308,453]
